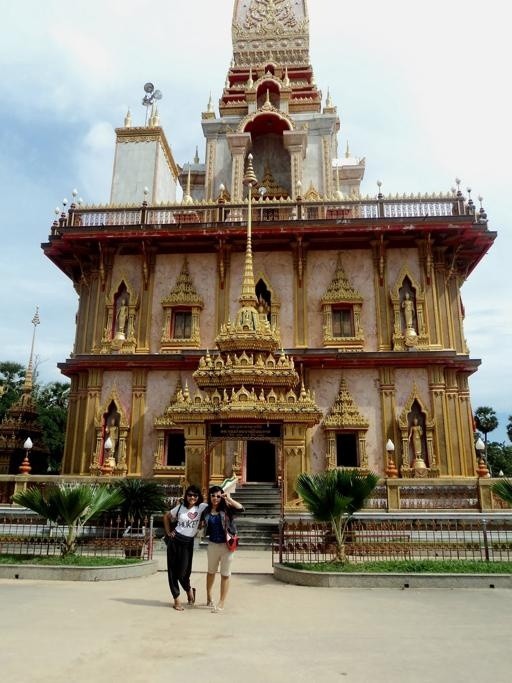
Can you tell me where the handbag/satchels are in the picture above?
[226,533,238,551]
[163,520,178,545]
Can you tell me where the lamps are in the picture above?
[377,178,383,199]
[100,437,113,475]
[218,183,225,204]
[451,176,487,220]
[296,179,302,200]
[19,436,32,474]
[385,438,399,479]
[53,189,83,226]
[475,437,490,478]
[142,186,149,207]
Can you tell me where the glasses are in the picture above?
[210,493,221,498]
[187,493,198,498]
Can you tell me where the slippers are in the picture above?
[211,606,224,613]
[174,605,183,610]
[188,588,196,607]
[207,602,214,607]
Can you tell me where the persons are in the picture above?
[163,485,233,610]
[409,418,424,459]
[200,486,245,608]
[402,293,415,329]
[105,415,118,457]
[116,299,128,332]
